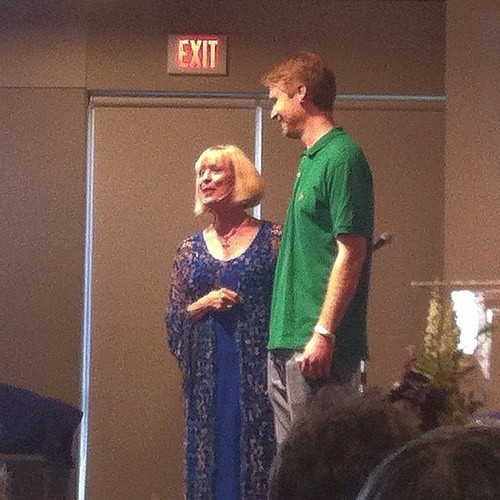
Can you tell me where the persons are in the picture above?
[260,52,374,451]
[165,144,283,500]
[270,385,500,500]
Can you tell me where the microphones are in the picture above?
[217,186,235,203]
[371,233,392,254]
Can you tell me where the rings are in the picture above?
[227,304,233,308]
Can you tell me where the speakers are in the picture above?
[0,382,84,455]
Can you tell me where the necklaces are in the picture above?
[212,214,253,249]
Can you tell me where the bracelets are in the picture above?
[314,325,337,341]
